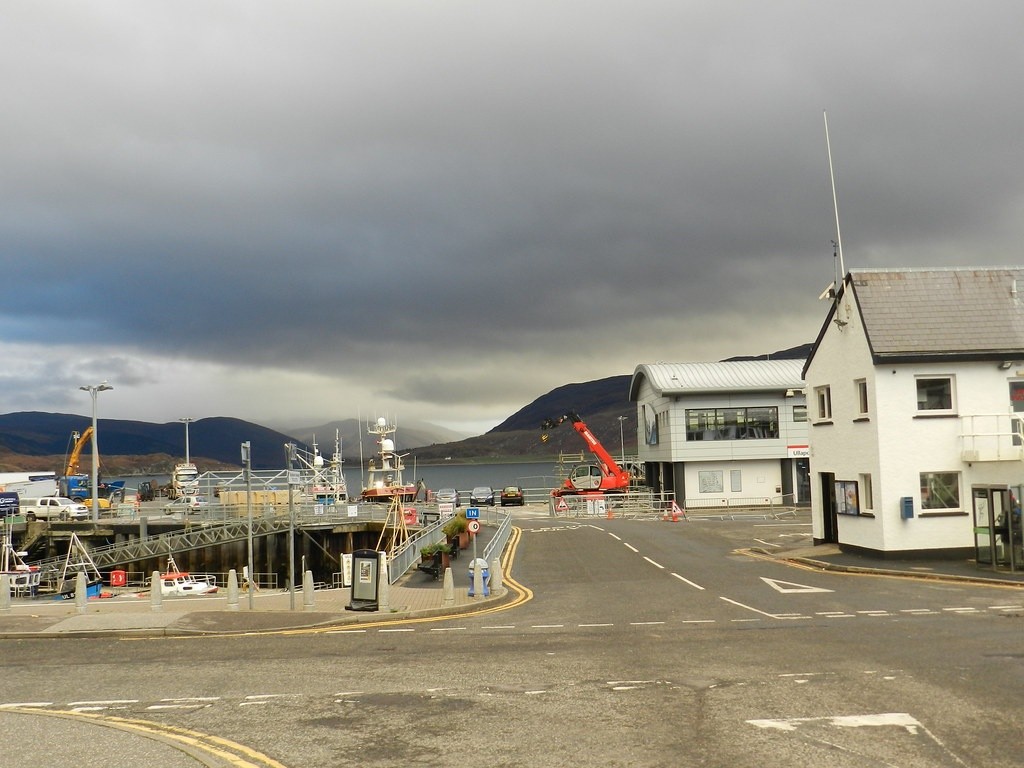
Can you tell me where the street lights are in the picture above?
[80,385,113,522]
[617,416,628,466]
[179,417,194,464]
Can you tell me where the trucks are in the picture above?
[0,475,90,503]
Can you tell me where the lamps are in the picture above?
[833,318,848,331]
[999,360,1013,368]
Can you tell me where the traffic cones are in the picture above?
[607,508,614,519]
[672,514,678,522]
[662,508,669,521]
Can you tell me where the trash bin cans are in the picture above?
[467,558,489,596]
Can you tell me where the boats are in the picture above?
[312,428,348,505]
[112,537,218,597]
[358,410,417,503]
[0,507,103,601]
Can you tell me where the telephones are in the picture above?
[997,511,1008,526]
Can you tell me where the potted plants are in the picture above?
[420,543,453,570]
[457,508,477,540]
[443,516,469,548]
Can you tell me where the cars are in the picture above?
[164,496,209,515]
[469,486,496,507]
[500,486,524,507]
[435,488,461,506]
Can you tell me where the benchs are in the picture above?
[418,559,438,580]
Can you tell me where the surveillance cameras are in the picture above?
[786,392,794,398]
[819,282,835,301]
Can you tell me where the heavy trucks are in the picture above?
[168,463,200,500]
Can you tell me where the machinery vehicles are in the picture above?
[66,428,100,476]
[541,412,630,509]
[137,482,155,502]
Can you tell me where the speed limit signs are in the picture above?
[468,520,480,533]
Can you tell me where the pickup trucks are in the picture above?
[19,497,89,522]
